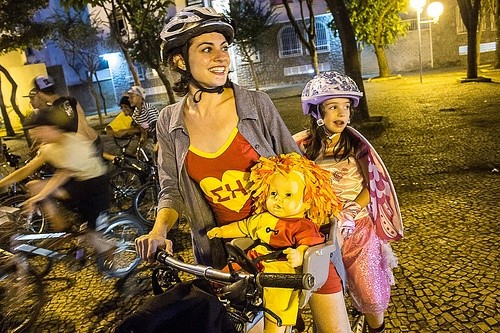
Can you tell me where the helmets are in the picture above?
[301,71,363,116]
[160,5,234,67]
[127,86,146,99]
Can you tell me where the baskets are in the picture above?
[108,311,244,333]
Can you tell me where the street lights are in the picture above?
[409,0,444,83]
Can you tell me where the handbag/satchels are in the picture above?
[115,266,239,333]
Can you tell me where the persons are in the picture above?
[0,76,163,267]
[134,3,353,333]
[207,151,345,333]
[289,71,404,333]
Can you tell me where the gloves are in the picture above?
[338,199,361,238]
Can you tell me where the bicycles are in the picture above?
[133,137,161,226]
[0,143,72,233]
[106,130,146,217]
[0,200,145,333]
[111,238,369,333]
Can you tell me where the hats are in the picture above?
[23,88,39,97]
[33,75,55,91]
